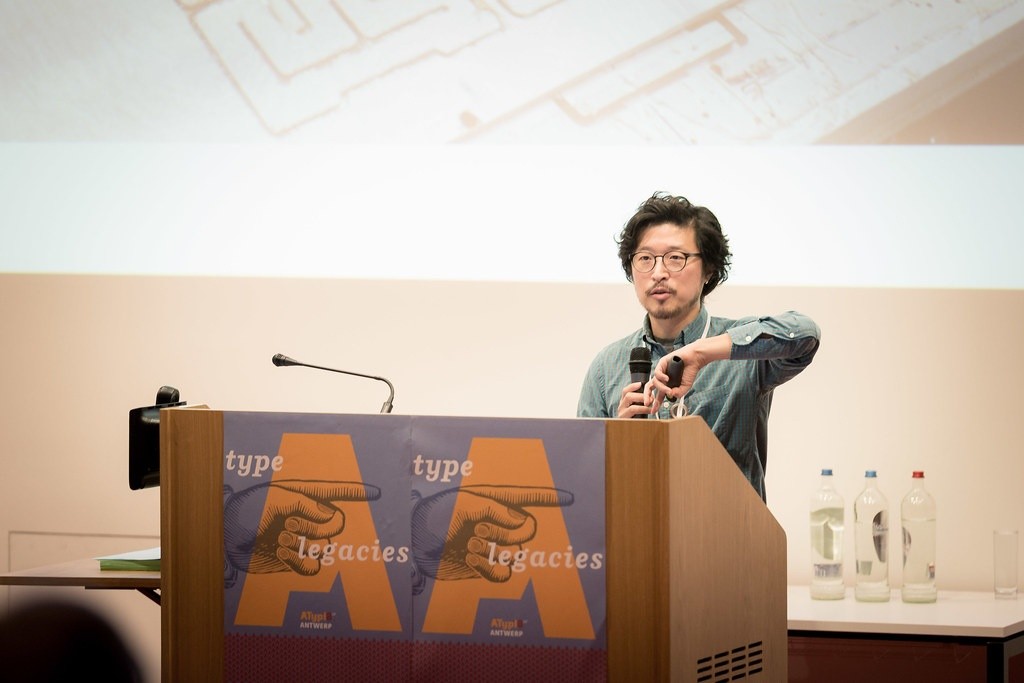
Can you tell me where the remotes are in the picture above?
[666,356,684,403]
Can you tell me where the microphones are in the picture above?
[629,347,653,420]
[272,353,394,414]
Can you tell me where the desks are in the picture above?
[0,560,1024,683]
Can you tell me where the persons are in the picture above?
[577,189,822,505]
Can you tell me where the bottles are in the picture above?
[899,472,939,603]
[854,470,891,602]
[809,468,846,600]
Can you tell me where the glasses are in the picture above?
[629,250,701,273]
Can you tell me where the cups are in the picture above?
[994,529,1018,599]
